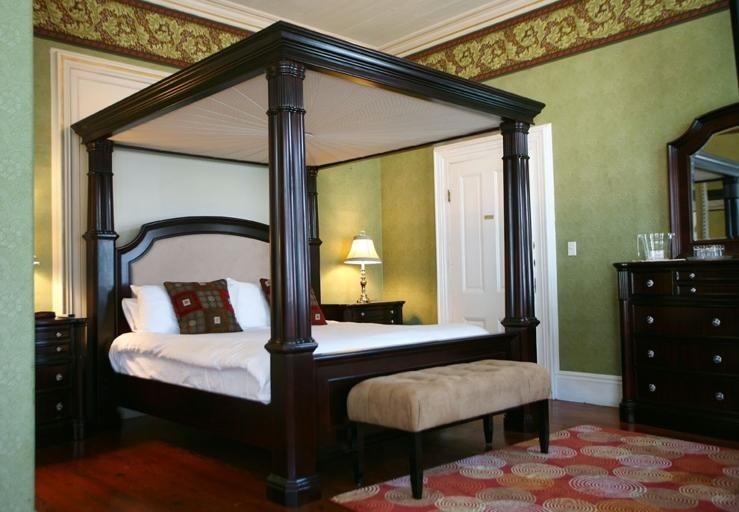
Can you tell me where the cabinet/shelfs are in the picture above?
[613,260,737,439]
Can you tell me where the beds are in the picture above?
[70,21,545,507]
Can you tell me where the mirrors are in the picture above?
[666,102,738,259]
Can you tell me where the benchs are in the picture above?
[346,358,553,500]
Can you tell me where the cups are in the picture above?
[693,245,725,257]
[632,231,679,263]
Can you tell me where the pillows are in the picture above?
[259,277,327,325]
[130,284,180,334]
[120,298,137,333]
[164,278,243,335]
[227,276,272,330]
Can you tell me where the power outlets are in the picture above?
[567,241,576,256]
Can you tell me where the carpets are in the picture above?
[330,425,734,511]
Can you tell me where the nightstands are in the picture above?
[322,301,407,326]
[35,317,93,465]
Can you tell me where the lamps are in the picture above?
[344,231,381,303]
